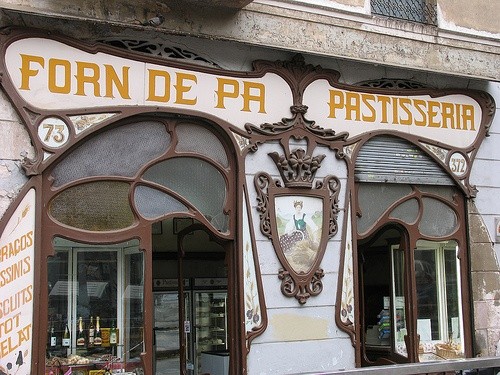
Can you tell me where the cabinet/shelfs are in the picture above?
[196,296,227,352]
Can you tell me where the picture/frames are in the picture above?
[152,221,163,235]
[173,218,194,234]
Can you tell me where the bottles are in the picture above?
[94,316,102,347]
[62,323,70,348]
[47,327,56,351]
[110,319,117,355]
[88,316,94,348]
[76,316,85,349]
